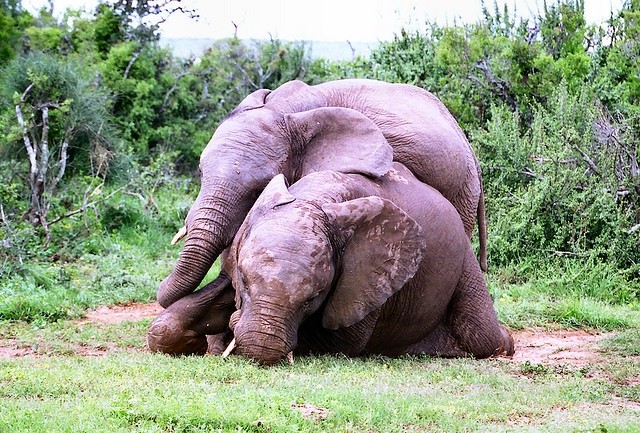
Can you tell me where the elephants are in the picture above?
[147,161,516,370]
[156,76,488,309]
